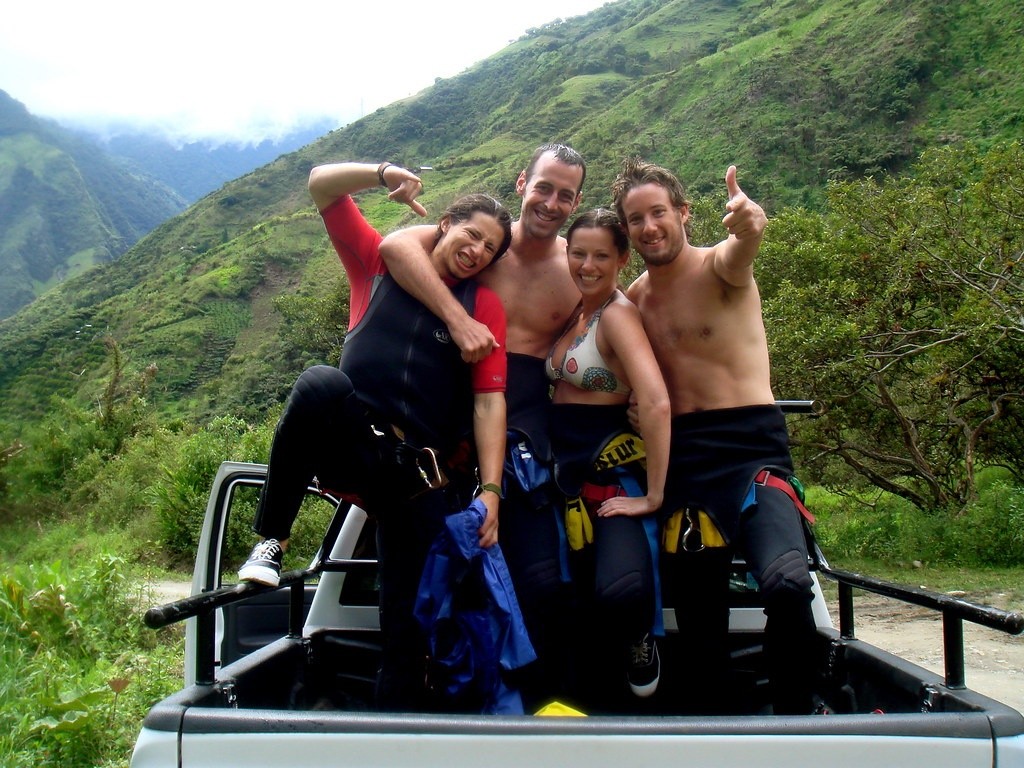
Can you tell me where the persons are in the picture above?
[240,160,506,716]
[379,143,820,715]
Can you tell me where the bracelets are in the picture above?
[481,483,503,500]
[379,162,392,186]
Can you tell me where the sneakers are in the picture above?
[626,632,661,696]
[238,539,284,586]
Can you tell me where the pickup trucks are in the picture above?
[128,460,1024,767]
